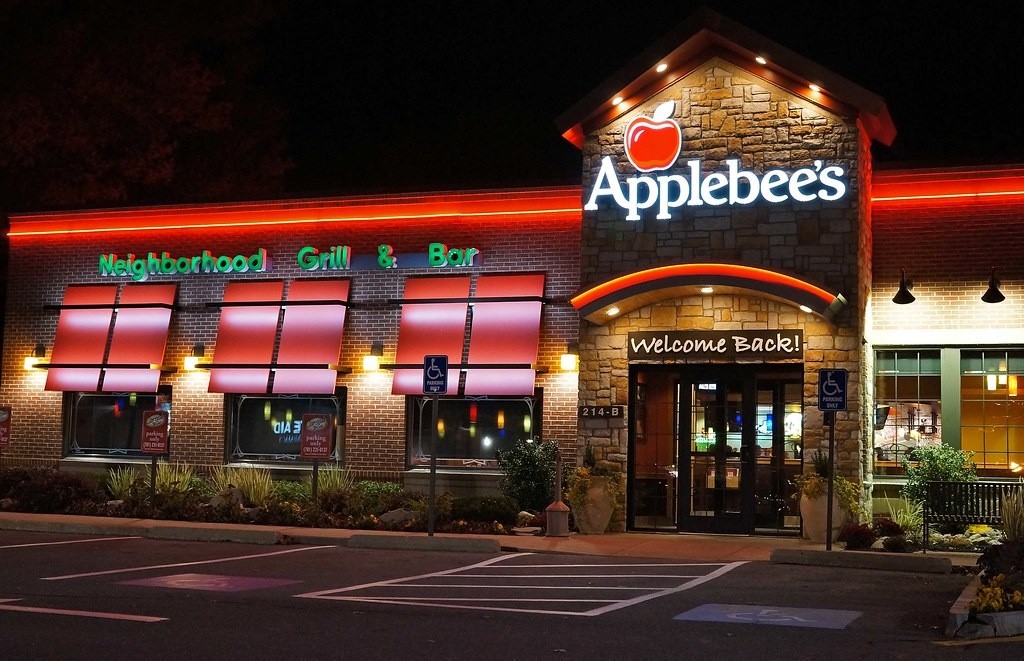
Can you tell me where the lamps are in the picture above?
[981,266,1005,303]
[363,344,384,371]
[892,268,916,305]
[560,343,579,371]
[24,347,46,370]
[184,345,204,370]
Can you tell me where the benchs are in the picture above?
[922,480,1024,554]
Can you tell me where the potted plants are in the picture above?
[564,440,623,535]
[792,448,873,544]
